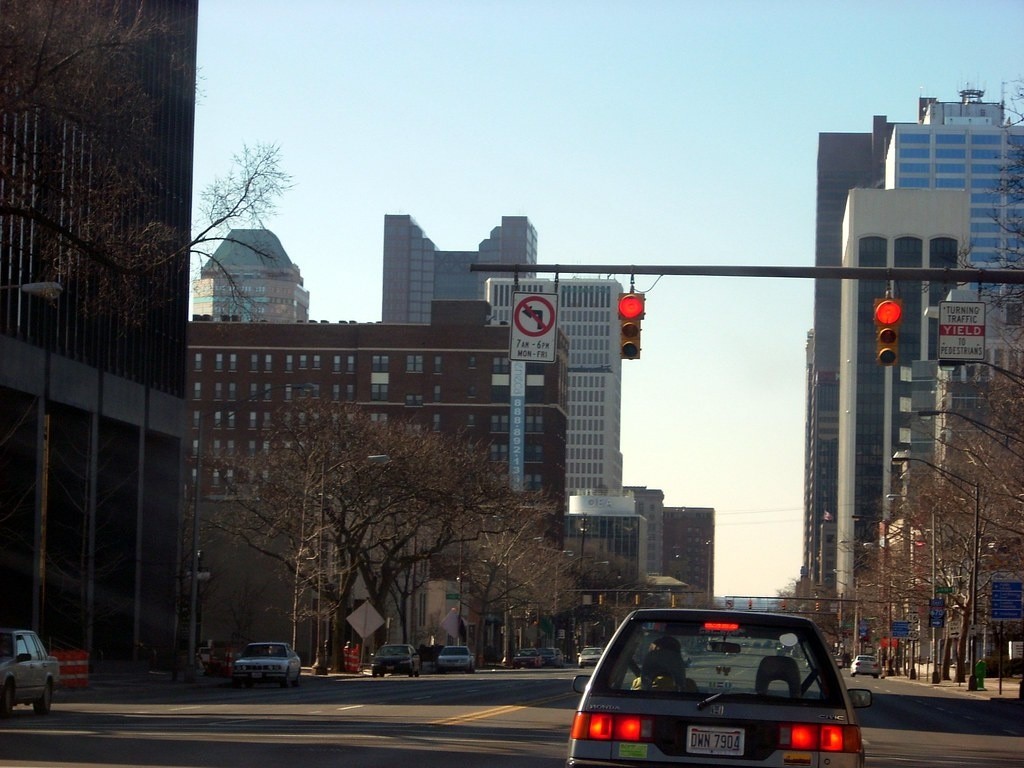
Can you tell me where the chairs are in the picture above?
[640,650,687,693]
[755,656,802,698]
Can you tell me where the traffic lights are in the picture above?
[749,603,753,611]
[875,298,905,367]
[782,603,786,611]
[616,292,646,360]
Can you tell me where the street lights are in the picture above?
[307,454,389,674]
[833,569,859,661]
[187,382,315,685]
[456,515,498,644]
[887,493,941,684]
[863,543,895,677]
[891,450,983,693]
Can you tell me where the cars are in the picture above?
[565,608,873,768]
[371,644,421,677]
[513,648,542,668]
[438,646,477,675]
[577,648,604,669]
[850,655,880,678]
[0,630,60,718]
[232,643,302,689]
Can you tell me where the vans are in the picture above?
[197,647,211,666]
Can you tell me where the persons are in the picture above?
[842,653,850,667]
[881,652,887,678]
[777,645,781,655]
[632,637,699,693]
[804,657,808,667]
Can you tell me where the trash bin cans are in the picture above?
[975,659,987,691]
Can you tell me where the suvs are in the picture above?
[540,648,566,668]
